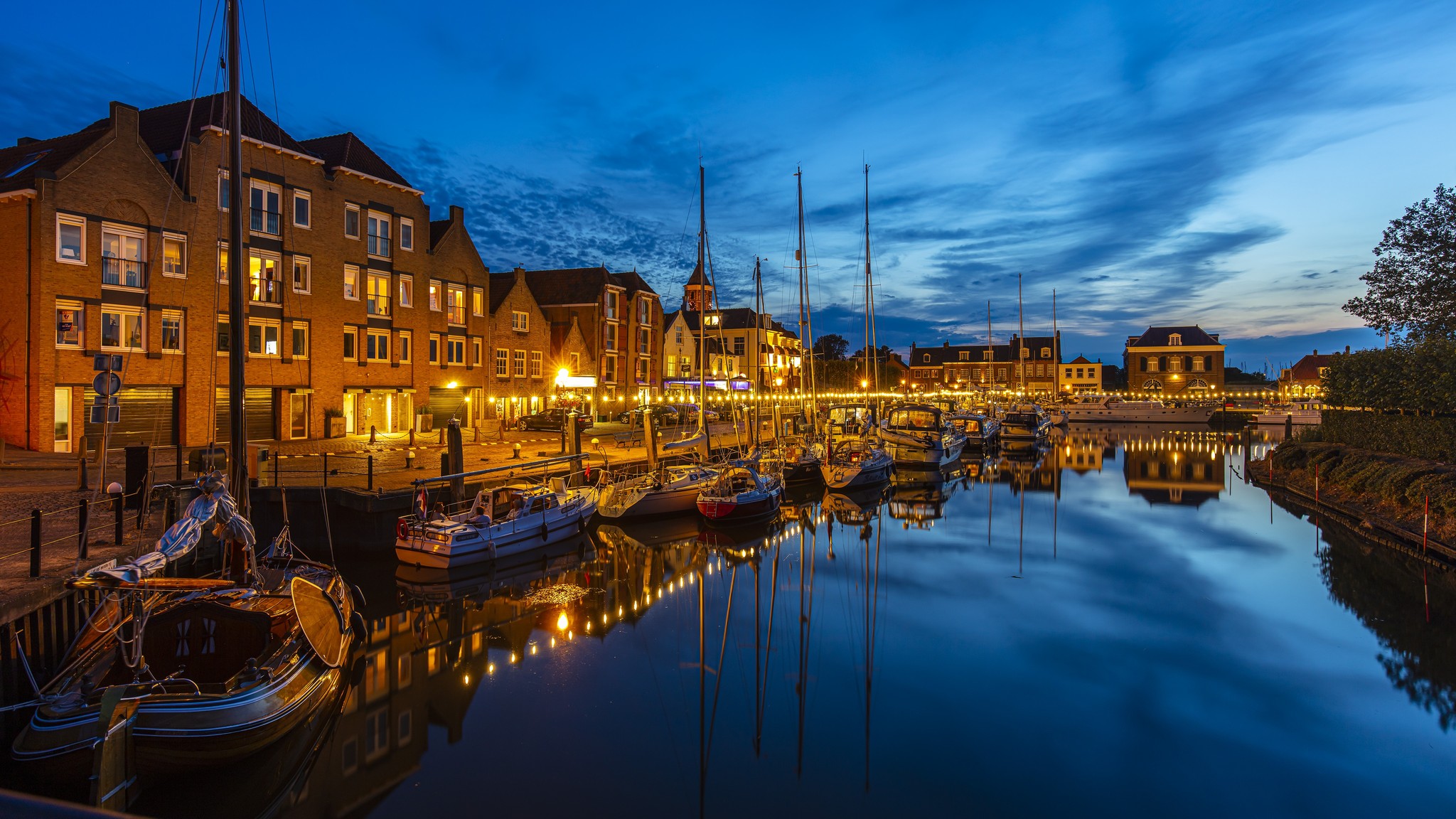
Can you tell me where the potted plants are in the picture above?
[414,403,434,433]
[320,405,348,438]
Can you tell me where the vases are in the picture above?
[1226,407,1234,409]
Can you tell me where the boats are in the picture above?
[1039,397,1222,423]
[889,466,1056,530]
[1247,398,1329,425]
[1266,404,1292,410]
[394,534,608,658]
[820,397,1056,467]
[696,466,785,528]
[396,452,602,568]
[695,516,787,553]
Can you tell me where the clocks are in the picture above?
[708,302,712,310]
[689,301,696,310]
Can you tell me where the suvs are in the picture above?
[658,403,702,425]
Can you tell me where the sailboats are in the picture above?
[2,1,374,795]
[601,146,785,518]
[1048,287,1069,430]
[620,496,885,819]
[747,154,899,493]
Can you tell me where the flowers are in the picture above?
[1225,404,1234,407]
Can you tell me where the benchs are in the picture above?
[613,431,644,448]
[1246,404,1260,409]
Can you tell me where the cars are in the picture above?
[617,404,679,426]
[698,405,720,423]
[515,407,593,433]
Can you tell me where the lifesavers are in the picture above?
[397,588,408,609]
[397,519,409,539]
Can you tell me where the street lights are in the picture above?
[1066,385,1070,393]
[955,385,958,393]
[902,380,905,392]
[912,384,916,395]
[1211,385,1216,404]
